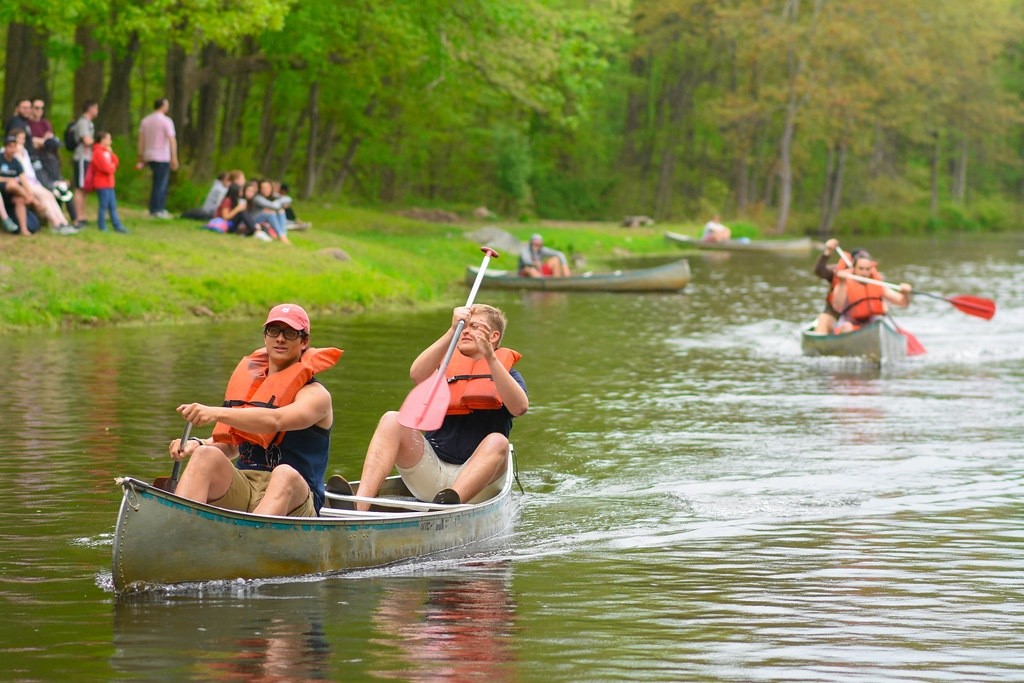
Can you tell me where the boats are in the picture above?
[468,259,691,293]
[801,318,908,368]
[112,443,514,592]
[665,231,814,259]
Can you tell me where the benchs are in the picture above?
[318,490,478,518]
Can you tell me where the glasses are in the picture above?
[266,325,301,340]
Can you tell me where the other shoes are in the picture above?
[254,230,273,242]
[3,217,18,233]
[325,474,357,511]
[50,223,79,235]
[145,209,175,221]
[427,488,460,512]
[294,222,312,232]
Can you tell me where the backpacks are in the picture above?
[83,161,93,192]
[64,122,82,153]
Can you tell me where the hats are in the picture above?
[534,239,542,245]
[4,135,17,147]
[54,182,73,202]
[263,303,310,335]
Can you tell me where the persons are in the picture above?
[136,97,179,218]
[201,170,313,243]
[168,304,333,516]
[0,98,126,237]
[325,303,528,512]
[812,238,912,334]
[518,233,571,277]
[703,213,730,242]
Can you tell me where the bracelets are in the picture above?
[137,154,143,162]
[189,436,204,445]
[15,177,20,182]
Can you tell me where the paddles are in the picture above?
[840,272,997,320]
[397,246,499,432]
[153,406,193,494]
[836,246,927,356]
